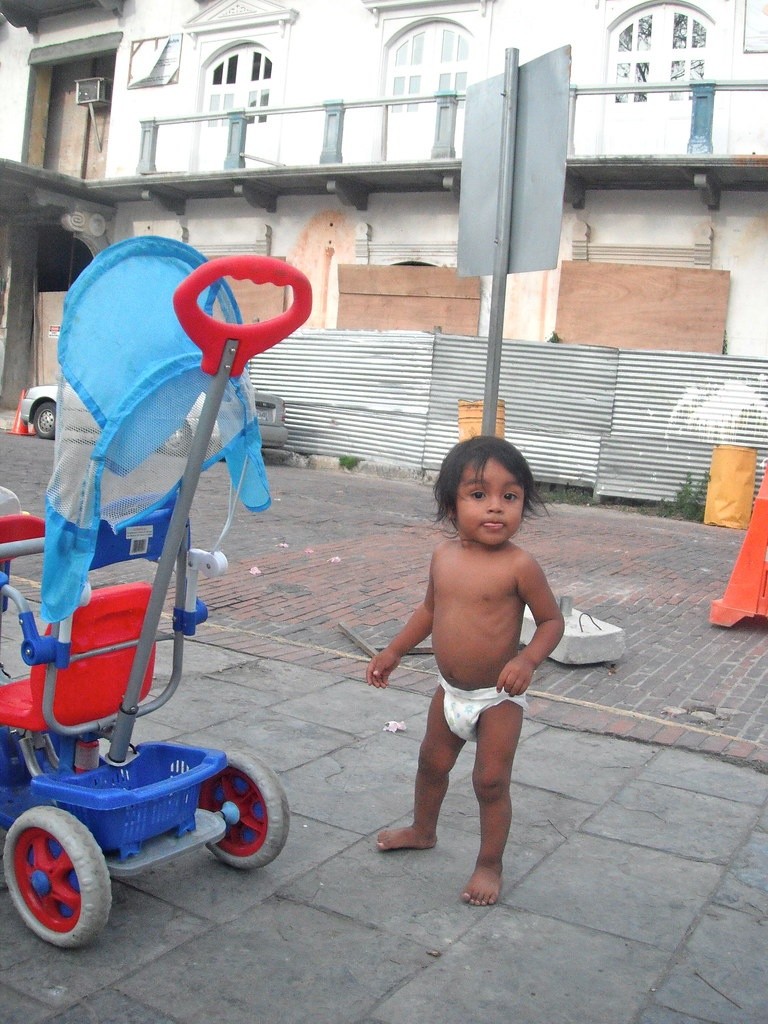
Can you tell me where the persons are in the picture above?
[364,434,566,906]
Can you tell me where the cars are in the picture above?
[20,379,289,456]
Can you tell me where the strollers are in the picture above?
[1,231,312,953]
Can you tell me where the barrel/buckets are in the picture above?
[457,400,505,442]
[704,445,758,530]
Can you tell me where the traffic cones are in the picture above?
[7,388,36,436]
[708,451,768,628]
